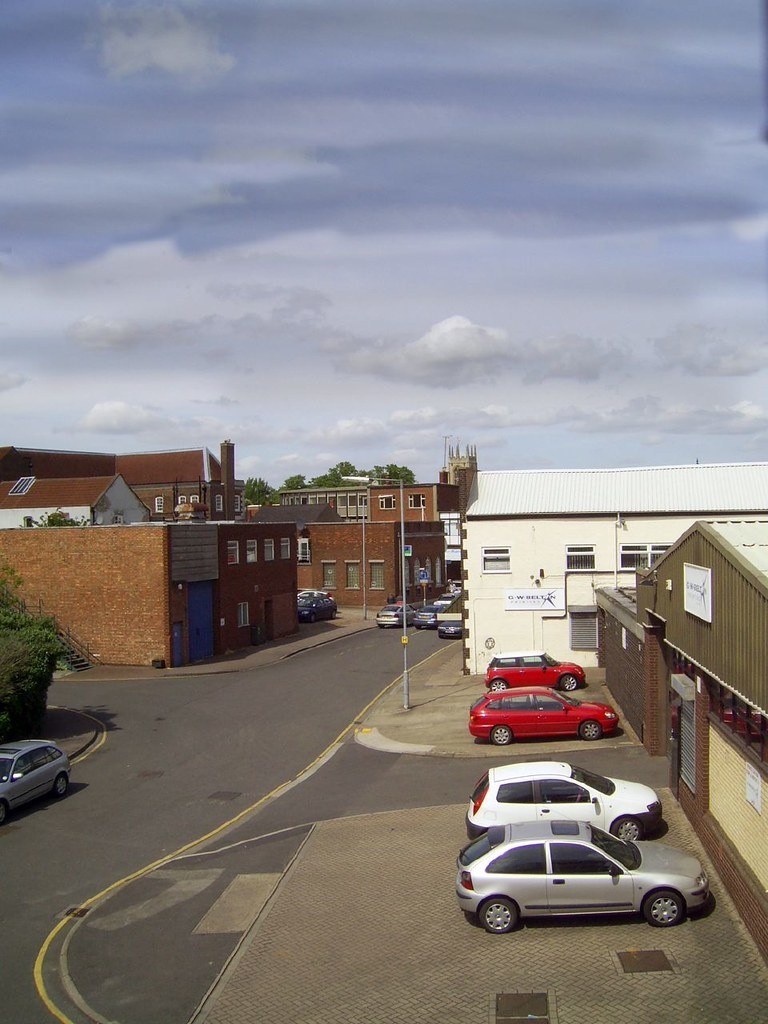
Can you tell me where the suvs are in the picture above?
[465,761,663,842]
[0,739,72,827]
[454,821,710,935]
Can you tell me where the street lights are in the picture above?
[342,476,411,710]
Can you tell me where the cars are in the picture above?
[484,651,587,692]
[438,621,464,639]
[296,591,338,623]
[433,579,463,607]
[377,603,419,629]
[469,685,620,747]
[412,605,444,630]
